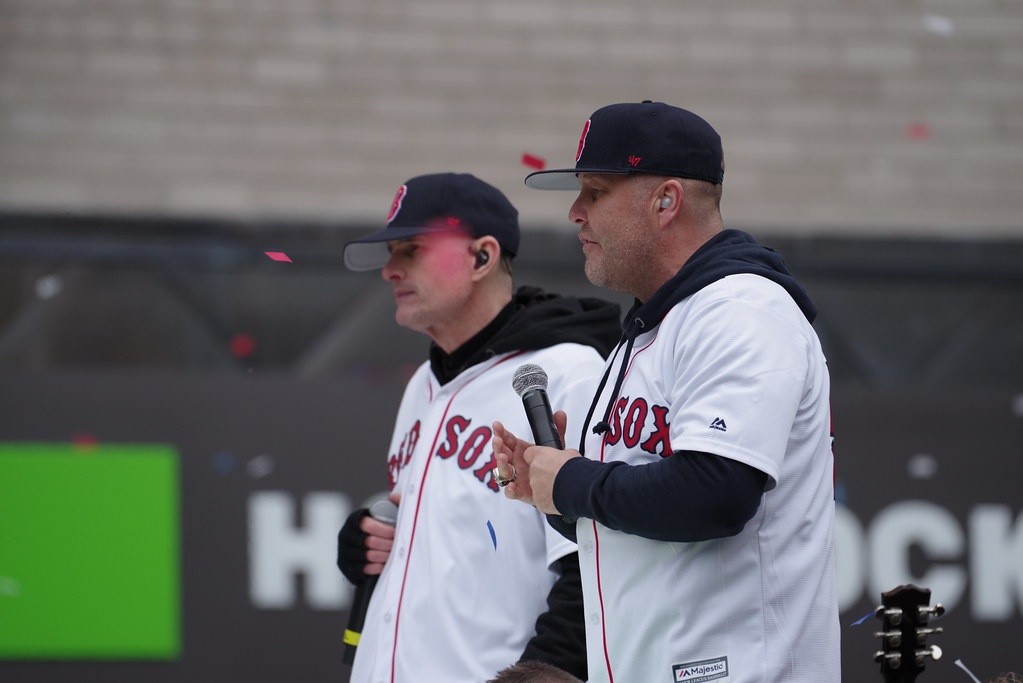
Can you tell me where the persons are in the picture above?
[336,171,623,683]
[491,100,843,683]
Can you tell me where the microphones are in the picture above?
[342,501,401,664]
[512,364,579,523]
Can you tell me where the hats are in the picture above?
[524,100,725,190]
[341,173,520,271]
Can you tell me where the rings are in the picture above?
[493,461,518,487]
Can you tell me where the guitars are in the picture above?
[872,583,945,683]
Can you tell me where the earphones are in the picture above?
[661,195,673,209]
[477,251,489,265]
